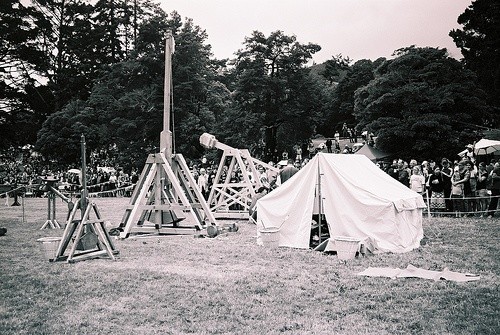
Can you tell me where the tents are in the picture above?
[256,152,427,257]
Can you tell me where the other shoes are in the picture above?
[422,209,494,219]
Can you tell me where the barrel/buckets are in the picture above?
[259,226,280,251]
[333,236,361,262]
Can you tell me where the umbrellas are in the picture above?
[457,137,500,164]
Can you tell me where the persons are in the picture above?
[0,144,154,198]
[293,122,390,171]
[484,119,490,132]
[462,143,477,165]
[374,156,500,219]
[490,119,496,132]
[165,153,299,211]
